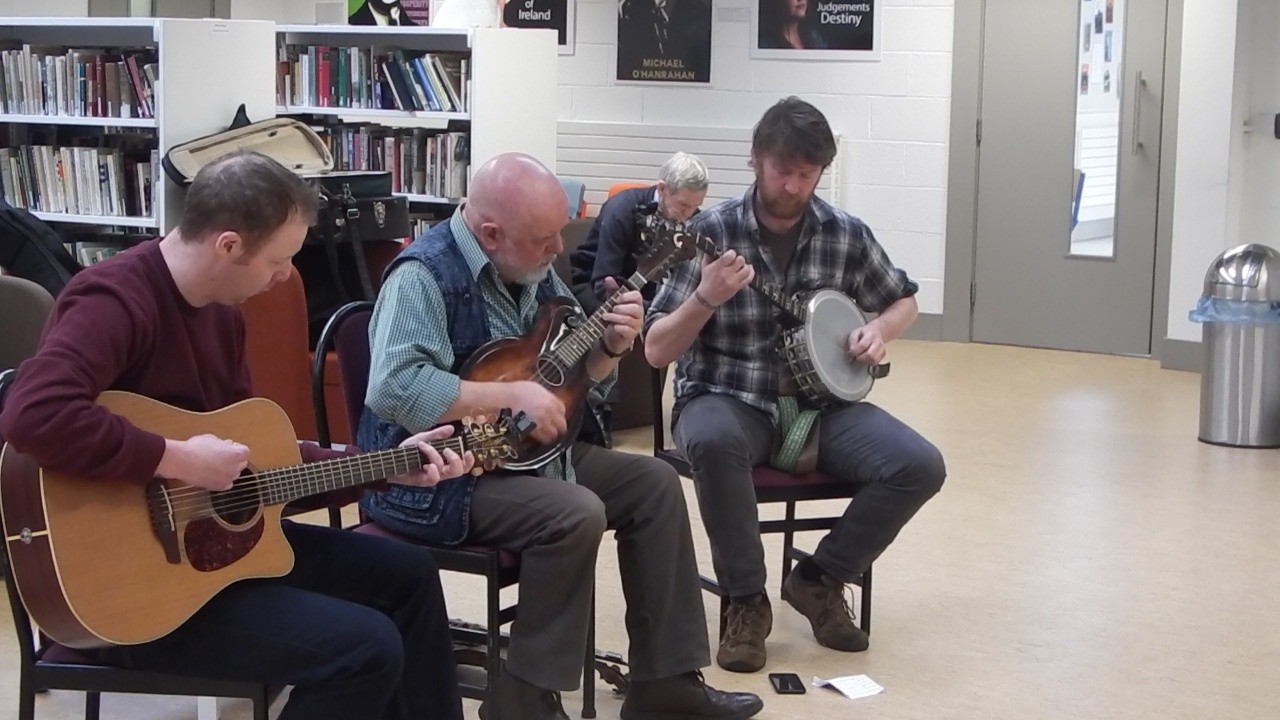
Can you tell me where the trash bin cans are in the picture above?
[1197,243,1280,448]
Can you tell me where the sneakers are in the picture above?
[780,557,869,652]
[717,587,773,673]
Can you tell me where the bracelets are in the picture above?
[694,289,719,311]
[600,335,634,358]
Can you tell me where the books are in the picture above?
[0,41,161,266]
[277,33,471,245]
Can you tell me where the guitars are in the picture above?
[636,200,877,410]
[0,390,520,651]
[459,226,698,472]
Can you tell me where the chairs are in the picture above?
[0,183,871,720]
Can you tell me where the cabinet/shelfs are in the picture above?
[271,25,558,237]
[0,16,276,266]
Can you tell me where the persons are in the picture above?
[568,150,711,451]
[641,93,947,674]
[357,153,764,720]
[0,152,464,720]
[758,0,827,49]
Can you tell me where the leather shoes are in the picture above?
[620,667,764,719]
[477,662,569,720]
